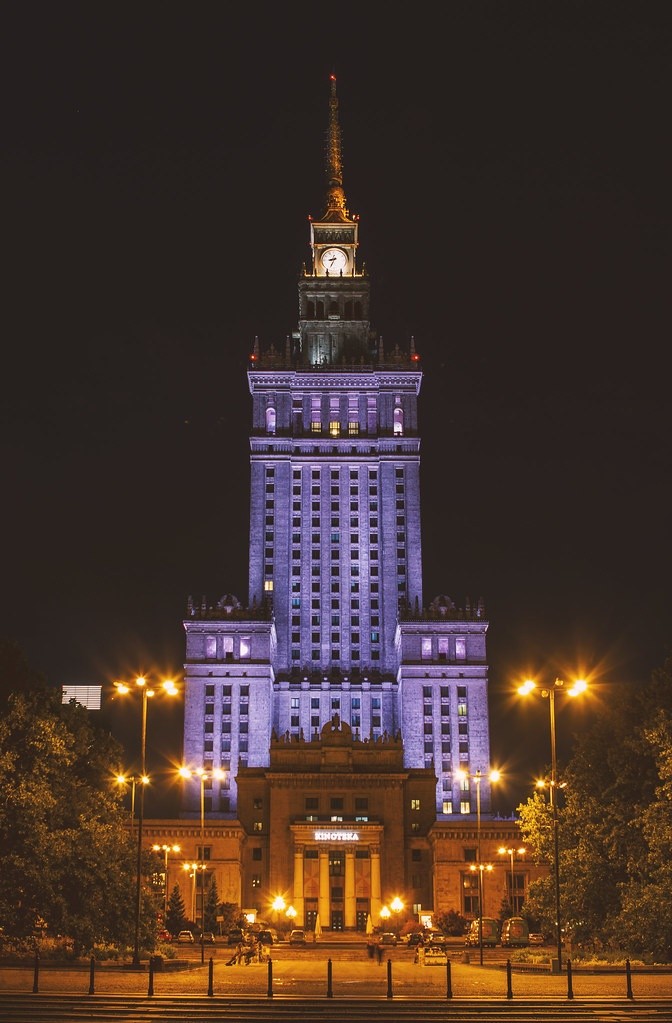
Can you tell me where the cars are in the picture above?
[528,934,543,946]
[379,933,397,946]
[408,934,424,946]
[199,932,215,944]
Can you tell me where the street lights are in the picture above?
[497,848,525,917]
[536,779,567,807]
[517,677,590,970]
[469,865,493,916]
[111,674,179,965]
[184,863,206,922]
[153,843,179,922]
[119,775,149,831]
[454,768,500,965]
[179,767,223,933]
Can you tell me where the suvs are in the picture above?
[157,930,172,943]
[178,931,194,944]
[290,930,306,945]
[258,930,273,945]
[429,933,446,947]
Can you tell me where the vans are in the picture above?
[501,917,529,948]
[228,928,247,944]
[466,917,499,947]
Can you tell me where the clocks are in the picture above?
[321,249,347,271]
